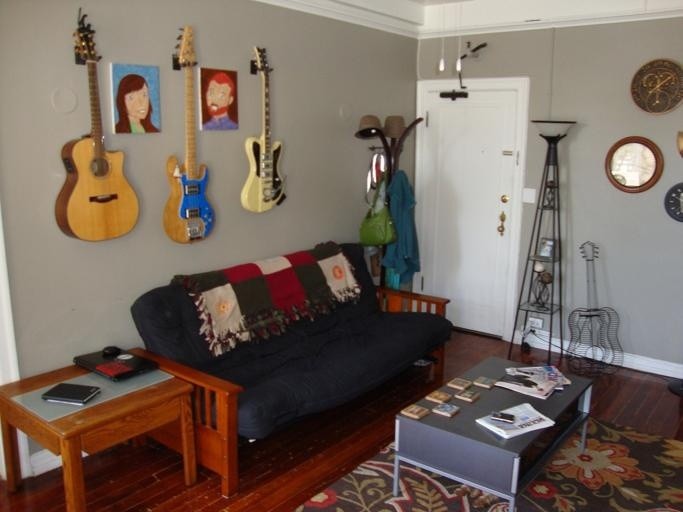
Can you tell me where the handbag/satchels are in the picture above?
[360,206,396,245]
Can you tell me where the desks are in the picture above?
[0,365,197,511]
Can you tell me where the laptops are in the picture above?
[73,349,159,382]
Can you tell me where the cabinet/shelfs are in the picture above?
[507,134,568,366]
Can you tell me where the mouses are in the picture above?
[103,346,120,356]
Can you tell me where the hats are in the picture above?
[382,116,410,139]
[355,115,382,139]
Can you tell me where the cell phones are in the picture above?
[490,410,515,424]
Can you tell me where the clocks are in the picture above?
[630,58,683,114]
[664,182,683,222]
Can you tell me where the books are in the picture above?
[494,365,572,400]
[41,383,101,404]
[474,402,557,440]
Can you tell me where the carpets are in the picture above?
[294,415,683,511]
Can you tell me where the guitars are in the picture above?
[240,45,287,213]
[567,241,624,377]
[54,6,139,241]
[163,25,213,243]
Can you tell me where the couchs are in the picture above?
[129,241,450,499]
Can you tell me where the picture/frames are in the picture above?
[605,136,664,193]
